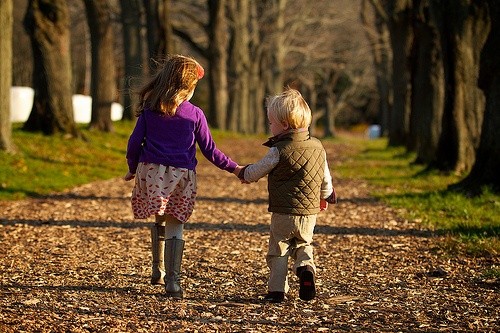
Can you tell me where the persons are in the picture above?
[123,55,246,299]
[237,88,337,304]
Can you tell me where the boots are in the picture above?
[150,226,165,285]
[163,235,185,297]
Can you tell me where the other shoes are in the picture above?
[264,292,284,303]
[299,265,316,301]
